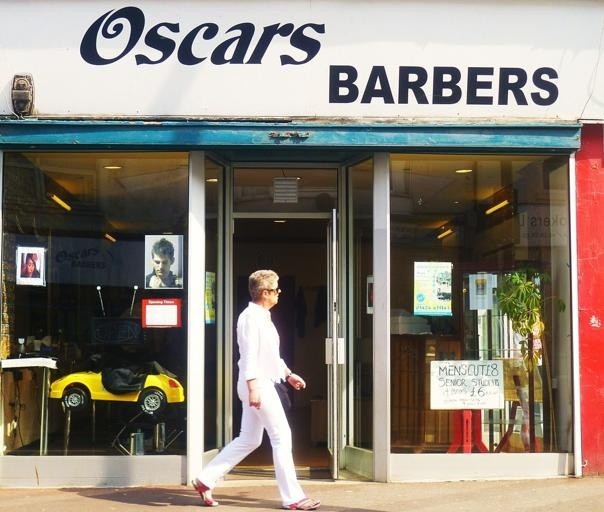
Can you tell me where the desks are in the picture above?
[0,358,58,455]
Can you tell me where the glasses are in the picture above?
[264,288,280,295]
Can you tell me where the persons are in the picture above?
[20,253,39,278]
[145,238,181,288]
[190,267,322,510]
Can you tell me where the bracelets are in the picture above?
[284,373,292,381]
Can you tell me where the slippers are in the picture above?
[192,477,219,507]
[282,497,321,510]
